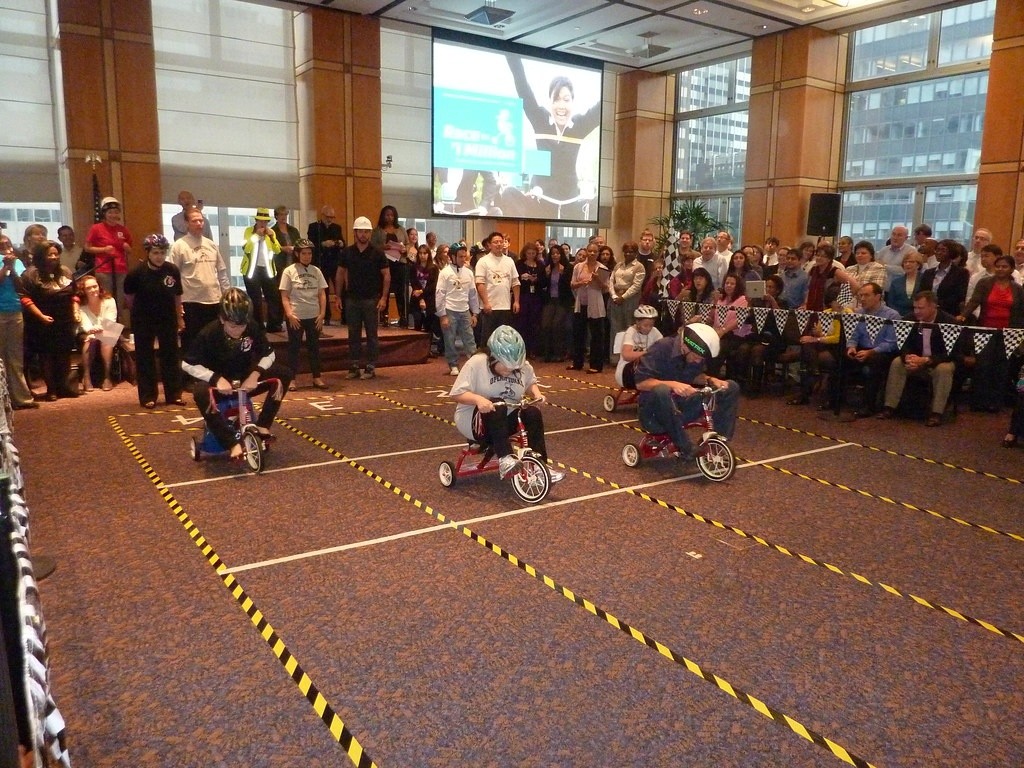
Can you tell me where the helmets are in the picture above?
[100,196,122,212]
[294,239,315,251]
[448,243,468,254]
[143,233,170,250]
[470,245,487,254]
[221,287,252,324]
[487,324,526,369]
[635,305,658,318]
[684,323,720,357]
[354,217,373,229]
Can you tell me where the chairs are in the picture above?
[776,360,965,422]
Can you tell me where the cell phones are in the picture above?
[197,200,203,208]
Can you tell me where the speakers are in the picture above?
[807,192,842,237]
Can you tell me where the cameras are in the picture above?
[387,155,392,159]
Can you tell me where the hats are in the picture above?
[255,207,271,221]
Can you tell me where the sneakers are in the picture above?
[549,469,566,483]
[499,454,523,482]
[450,366,459,376]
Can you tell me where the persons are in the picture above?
[182,288,293,461]
[434,54,600,223]
[634,323,739,471]
[370,206,410,328]
[786,223,1024,446]
[448,325,567,487]
[645,231,815,397]
[335,217,391,380]
[241,207,281,333]
[307,206,347,325]
[170,208,232,393]
[270,205,301,327]
[406,228,573,376]
[84,199,134,321]
[1,224,118,405]
[614,305,664,388]
[565,228,657,374]
[125,236,188,407]
[172,191,213,242]
[280,239,330,391]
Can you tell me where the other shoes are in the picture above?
[734,374,944,428]
[289,381,297,391]
[231,450,243,466]
[526,347,609,374]
[681,445,705,460]
[362,367,376,379]
[145,400,189,408]
[346,370,360,379]
[313,382,328,389]
[15,378,112,411]
[260,431,277,445]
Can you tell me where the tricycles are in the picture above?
[603,385,640,413]
[190,377,284,474]
[621,385,736,482]
[438,396,552,503]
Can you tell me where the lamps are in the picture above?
[463,0,514,26]
[626,30,671,60]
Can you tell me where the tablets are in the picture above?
[745,281,766,298]
